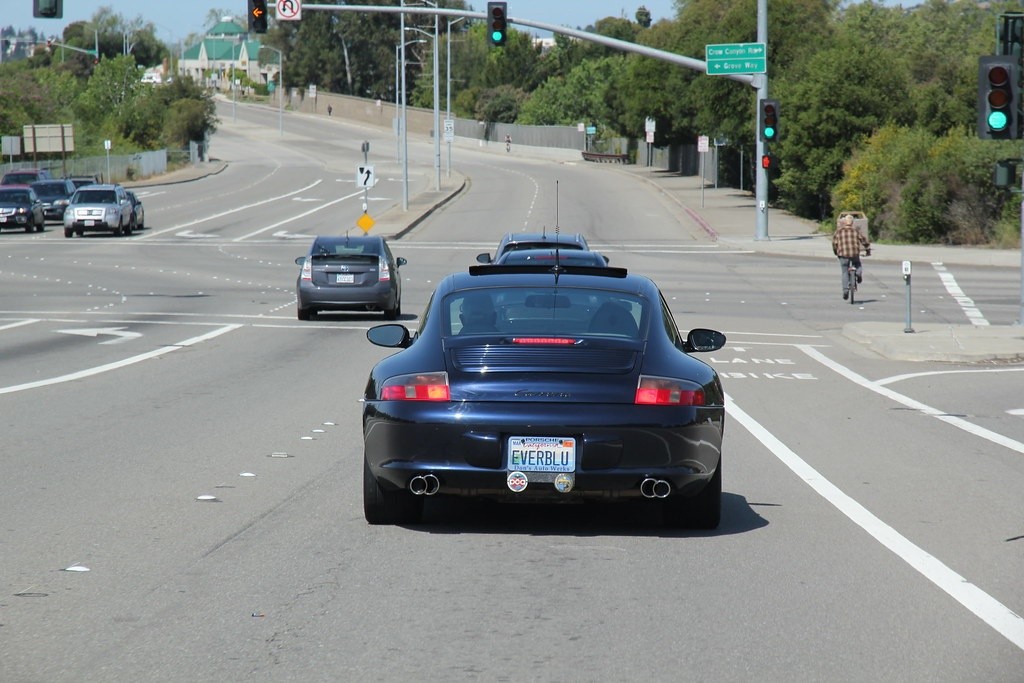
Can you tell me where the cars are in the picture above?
[294,235,408,321]
[459,248,632,331]
[0,187,45,233]
[475,232,610,264]
[0,170,105,220]
[63,184,144,238]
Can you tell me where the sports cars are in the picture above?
[360,262,727,529]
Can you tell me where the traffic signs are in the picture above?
[705,43,766,76]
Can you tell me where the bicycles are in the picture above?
[844,253,871,304]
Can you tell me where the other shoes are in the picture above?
[856,273,862,284]
[843,291,849,300]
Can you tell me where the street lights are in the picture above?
[259,45,283,141]
[395,39,427,164]
[404,27,436,153]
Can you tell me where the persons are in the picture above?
[832,214,872,300]
[458,293,500,334]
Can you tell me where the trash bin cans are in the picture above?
[837,211,870,252]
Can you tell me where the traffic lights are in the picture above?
[93,58,99,65]
[248,0,268,34]
[976,53,1019,140]
[47,41,50,47]
[762,155,777,169]
[487,2,508,45]
[759,98,779,143]
